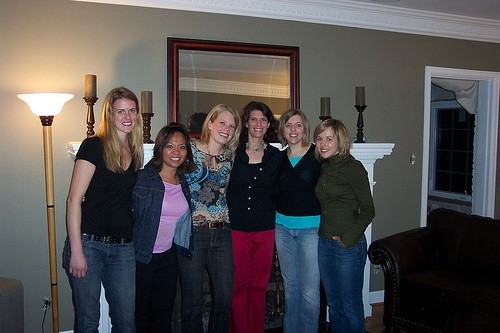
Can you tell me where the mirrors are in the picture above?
[167,36,300,141]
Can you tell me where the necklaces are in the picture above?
[206,141,224,171]
[246,142,266,153]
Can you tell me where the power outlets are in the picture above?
[41,296,53,312]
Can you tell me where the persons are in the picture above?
[62,87,144,333]
[82,122,198,333]
[226,101,279,333]
[176,104,241,333]
[313,118,375,333]
[275,110,323,333]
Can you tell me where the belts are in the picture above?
[89,234,133,244]
[193,220,228,229]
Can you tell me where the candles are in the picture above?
[141,90,152,113]
[355,87,366,105]
[84,74,97,98]
[320,97,330,116]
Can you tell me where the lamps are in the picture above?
[17,93,75,333]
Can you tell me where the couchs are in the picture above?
[368,208,500,333]
